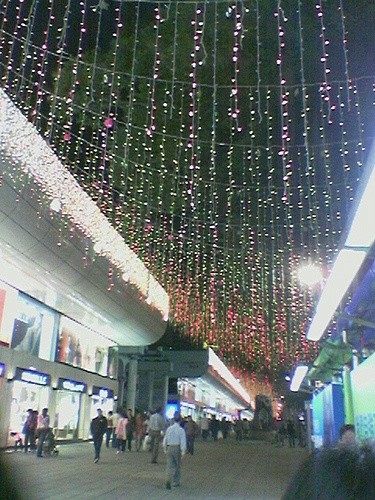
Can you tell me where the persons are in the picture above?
[90,408,374,500]
[11,408,50,457]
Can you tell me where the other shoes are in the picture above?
[165,483,171,489]
[172,484,180,487]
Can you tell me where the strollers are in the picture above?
[40,428,58,457]
